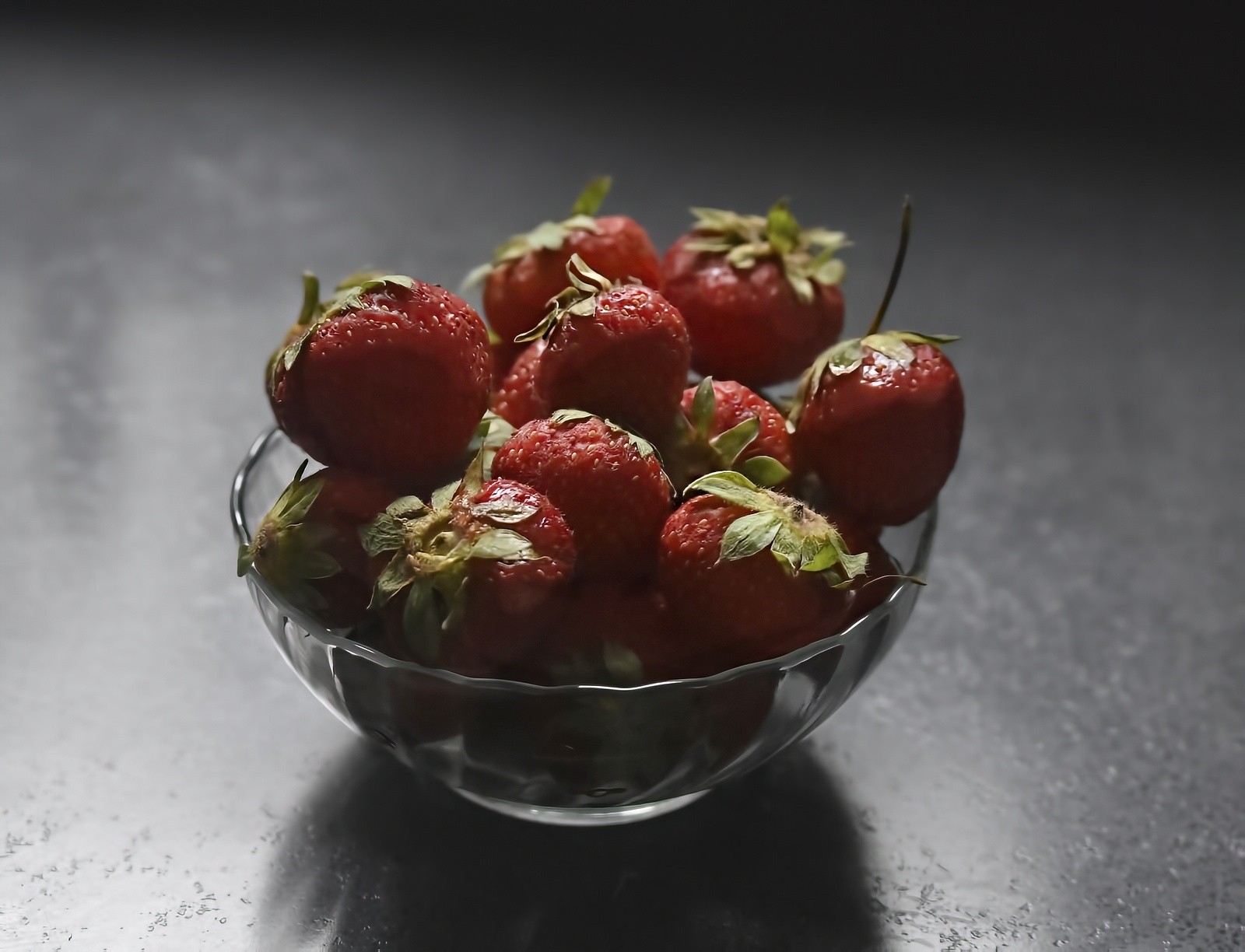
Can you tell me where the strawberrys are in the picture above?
[239,175,966,755]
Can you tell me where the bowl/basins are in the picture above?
[229,423,949,829]
